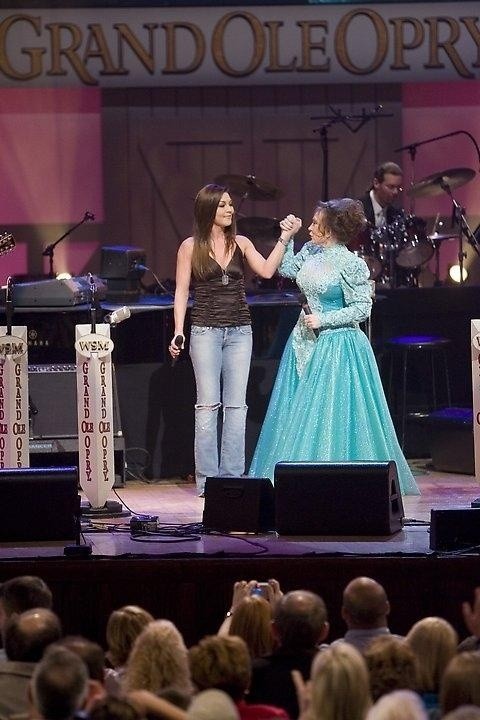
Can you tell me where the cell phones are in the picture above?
[251,582,269,596]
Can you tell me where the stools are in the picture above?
[381,332,456,457]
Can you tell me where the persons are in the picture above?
[218,576,428,718]
[103,605,289,718]
[278,237,288,246]
[277,193,376,464]
[353,161,405,254]
[167,180,303,498]
[405,588,479,718]
[0,576,106,719]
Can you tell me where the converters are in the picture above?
[130,515,159,532]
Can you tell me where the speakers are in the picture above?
[203,477,273,532]
[274,459,404,536]
[429,406,474,474]
[0,466,79,542]
[430,508,480,550]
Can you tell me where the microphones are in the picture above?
[297,293,319,338]
[171,335,183,367]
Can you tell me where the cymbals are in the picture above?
[234,216,281,238]
[214,173,283,202]
[429,231,456,241]
[408,167,477,198]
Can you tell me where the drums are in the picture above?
[396,238,435,267]
